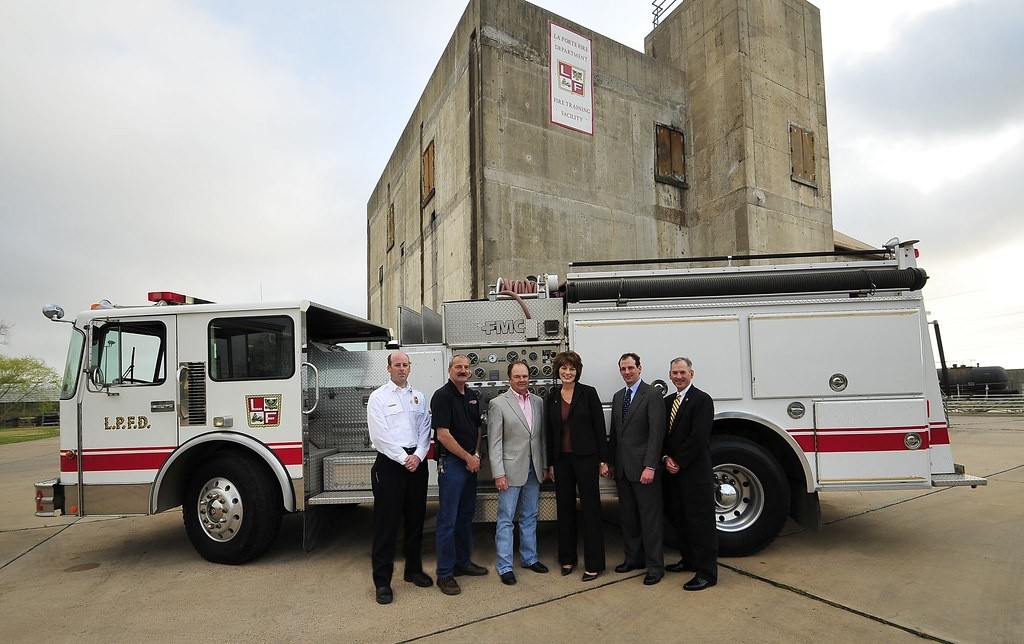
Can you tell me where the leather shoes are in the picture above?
[683,576,714,591]
[562,565,573,576]
[523,561,548,573]
[615,561,633,573]
[501,571,517,585]
[582,573,597,580]
[665,562,685,572]
[643,572,664,585]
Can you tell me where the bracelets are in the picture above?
[662,455,667,462]
[599,462,606,466]
[474,453,481,459]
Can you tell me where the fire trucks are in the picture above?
[33,236,987,557]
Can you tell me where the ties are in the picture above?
[622,389,633,427]
[669,394,681,432]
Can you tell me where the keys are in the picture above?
[436,463,445,473]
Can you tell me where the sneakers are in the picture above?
[404,572,433,587]
[454,562,488,576]
[376,585,393,604]
[436,576,461,595]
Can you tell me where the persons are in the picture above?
[367,352,434,605]
[543,350,610,582]
[430,354,489,596]
[488,361,548,585]
[610,353,667,586]
[664,357,718,590]
